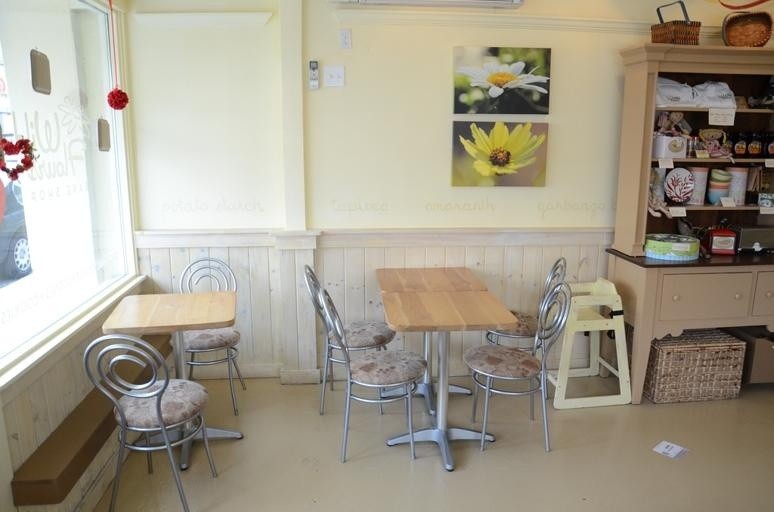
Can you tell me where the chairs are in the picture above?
[302,262,399,417]
[313,283,430,464]
[461,283,572,453]
[80,330,217,511]
[482,256,568,401]
[174,258,246,418]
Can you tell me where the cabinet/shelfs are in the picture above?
[608,44,774,256]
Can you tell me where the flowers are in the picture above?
[0,139,38,180]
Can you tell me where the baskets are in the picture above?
[651,0,701,46]
[723,12,773,47]
[628,326,746,403]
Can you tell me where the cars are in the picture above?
[0,110,32,280]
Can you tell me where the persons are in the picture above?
[656,110,736,166]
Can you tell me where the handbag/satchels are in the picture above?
[656,76,737,108]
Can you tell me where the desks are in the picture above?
[603,248,773,404]
[102,294,244,469]
[374,268,519,473]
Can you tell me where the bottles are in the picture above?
[685,134,700,158]
[733,130,774,158]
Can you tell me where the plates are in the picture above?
[663,168,695,203]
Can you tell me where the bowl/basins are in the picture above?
[709,168,732,205]
